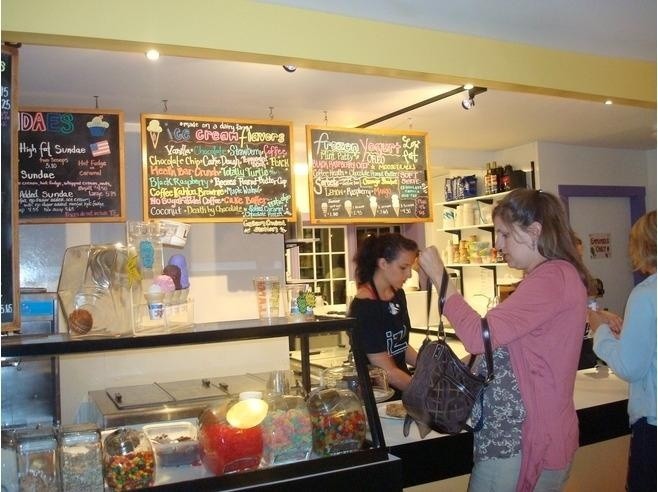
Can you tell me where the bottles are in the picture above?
[484,162,493,194]
[491,159,501,193]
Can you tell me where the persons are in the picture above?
[345,234,418,402]
[588,209,657,491]
[571,236,623,334]
[593,278,606,311]
[418,190,599,491]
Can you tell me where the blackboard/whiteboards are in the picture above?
[17,106,128,223]
[2,43,21,333]
[140,113,297,223]
[306,125,434,224]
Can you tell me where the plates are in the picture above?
[377,399,408,420]
[374,386,396,403]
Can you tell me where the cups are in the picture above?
[253,275,281,320]
[144,288,190,320]
[443,202,480,229]
[280,284,307,318]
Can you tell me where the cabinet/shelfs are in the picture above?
[436,160,535,338]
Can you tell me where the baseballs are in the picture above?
[68,309,93,336]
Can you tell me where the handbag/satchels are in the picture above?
[402,268,494,438]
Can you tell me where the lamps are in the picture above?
[462,87,486,110]
[284,65,296,73]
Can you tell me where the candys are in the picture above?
[2,433,61,489]
[262,409,313,463]
[103,449,156,489]
[199,421,263,473]
[311,411,367,456]
[58,440,105,491]
